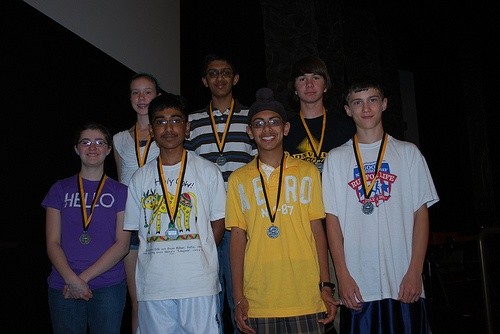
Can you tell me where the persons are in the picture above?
[281,56,355,334]
[40,122,132,334]
[114,74,163,334]
[322,79,439,334]
[184,57,258,334]
[123,94,229,334]
[227,99,337,334]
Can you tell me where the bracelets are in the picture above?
[233,295,245,309]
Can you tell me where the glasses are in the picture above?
[250,119,284,127]
[205,70,232,77]
[77,139,108,146]
[152,118,184,127]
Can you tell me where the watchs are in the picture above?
[319,281,336,296]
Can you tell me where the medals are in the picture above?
[166,227,178,240]
[317,164,324,173]
[268,226,280,238]
[80,232,91,245]
[216,157,225,164]
[362,203,375,215]
